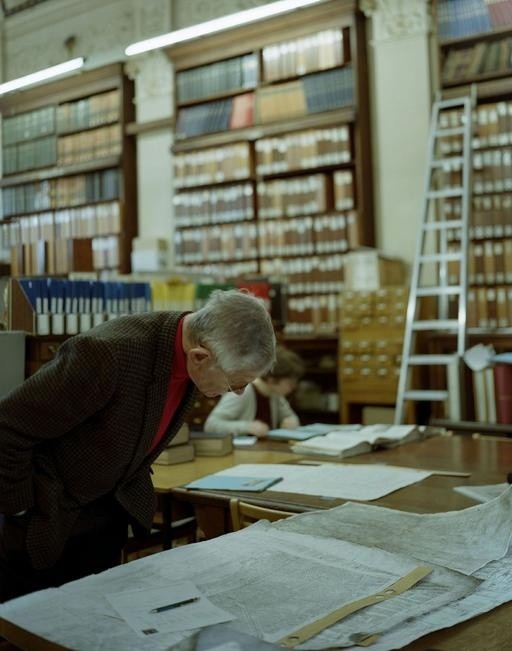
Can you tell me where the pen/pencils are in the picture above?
[150,597,201,614]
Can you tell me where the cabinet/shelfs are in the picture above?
[25,319,287,433]
[160,0,375,423]
[335,287,411,426]
[2,61,136,335]
[421,1,512,441]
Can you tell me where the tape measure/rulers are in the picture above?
[298,460,471,477]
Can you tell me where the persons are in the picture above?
[204,344,305,439]
[0,289,277,606]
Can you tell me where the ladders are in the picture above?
[394,96,470,425]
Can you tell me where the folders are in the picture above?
[19,279,152,314]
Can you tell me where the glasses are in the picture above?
[218,363,250,393]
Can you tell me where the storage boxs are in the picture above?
[342,249,408,291]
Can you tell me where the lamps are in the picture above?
[1,34,88,94]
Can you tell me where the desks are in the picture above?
[0,438,509,651]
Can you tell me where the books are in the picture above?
[172,26,359,335]
[439,38,512,327]
[265,422,419,458]
[459,344,512,428]
[1,89,120,276]
[16,276,270,336]
